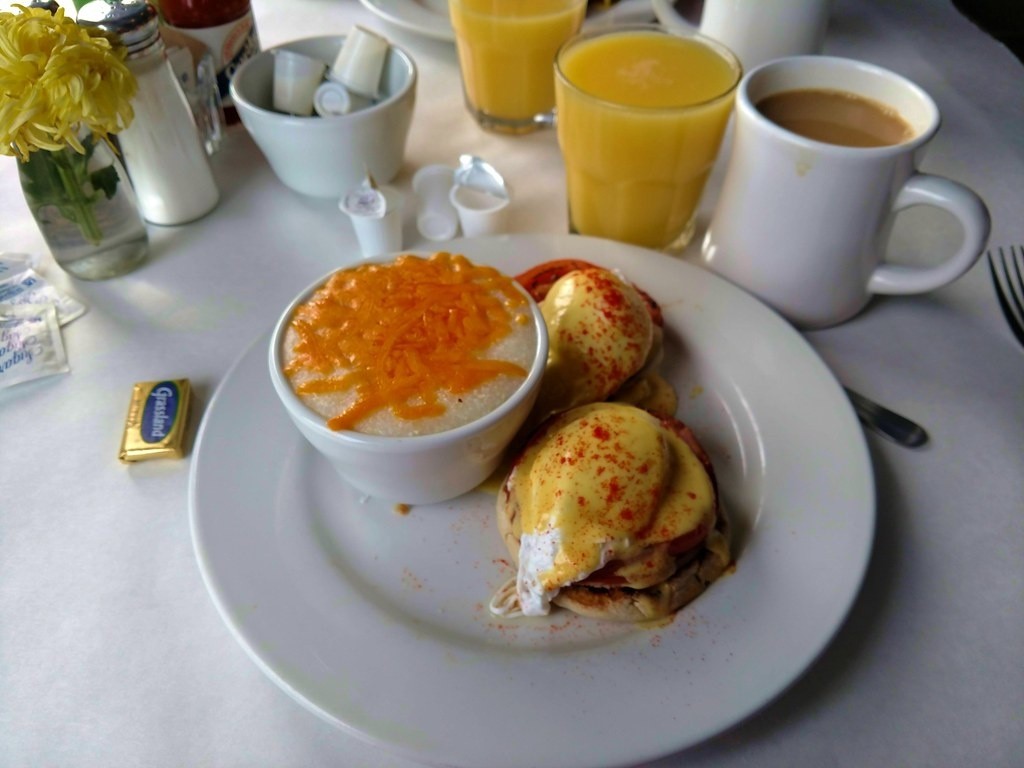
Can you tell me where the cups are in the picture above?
[271,24,390,118]
[702,56,990,329]
[553,22,743,255]
[343,168,509,256]
[651,0,831,75]
[449,0,588,135]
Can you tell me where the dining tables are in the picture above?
[0,0,1024,768]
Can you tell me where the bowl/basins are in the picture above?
[269,251,550,505]
[229,33,417,201]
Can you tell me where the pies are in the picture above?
[495,404,732,623]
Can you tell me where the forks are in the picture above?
[986,245,1024,351]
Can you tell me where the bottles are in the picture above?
[75,0,221,226]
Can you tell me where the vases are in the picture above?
[16,123,152,280]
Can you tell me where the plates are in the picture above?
[359,0,455,41]
[189,235,877,768]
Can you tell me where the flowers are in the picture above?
[0,4,139,247]
